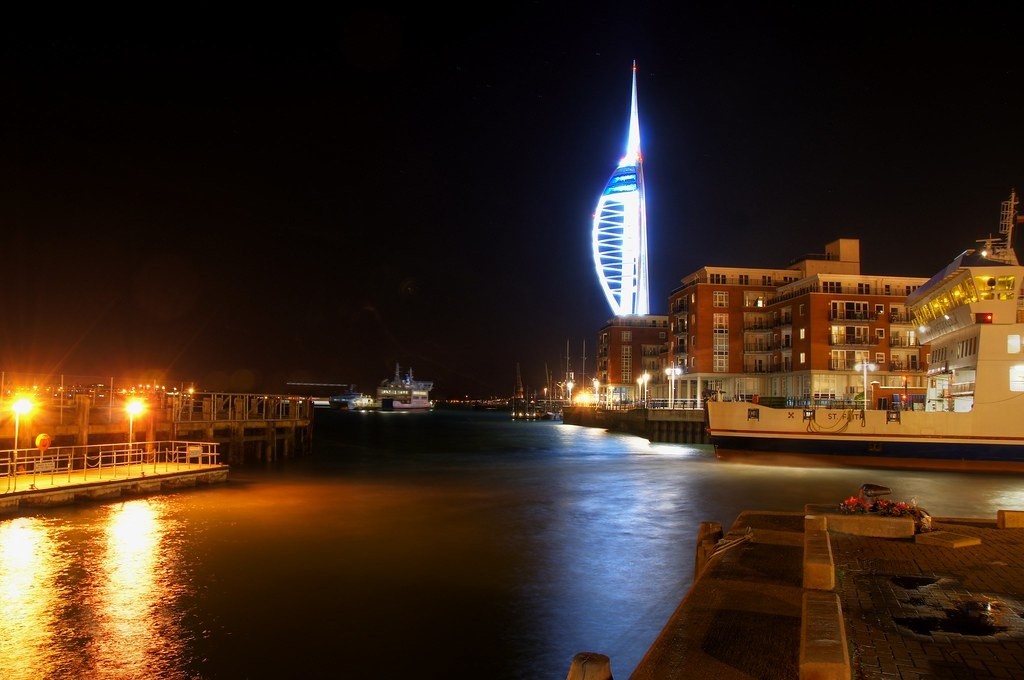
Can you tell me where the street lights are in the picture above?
[643,370,650,408]
[14,400,30,474]
[855,360,875,410]
[666,362,681,409]
[568,381,573,406]
[637,375,643,401]
[127,401,143,465]
[595,380,599,406]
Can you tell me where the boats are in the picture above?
[328,363,434,410]
[705,192,1024,476]
